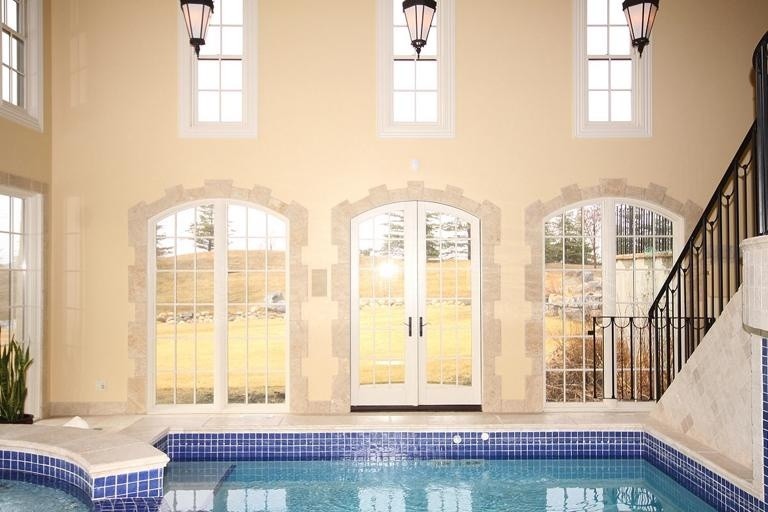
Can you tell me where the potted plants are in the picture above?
[1,335,40,427]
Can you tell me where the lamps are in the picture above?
[403,0,437,57]
[180,0,213,55]
[621,0,660,59]
